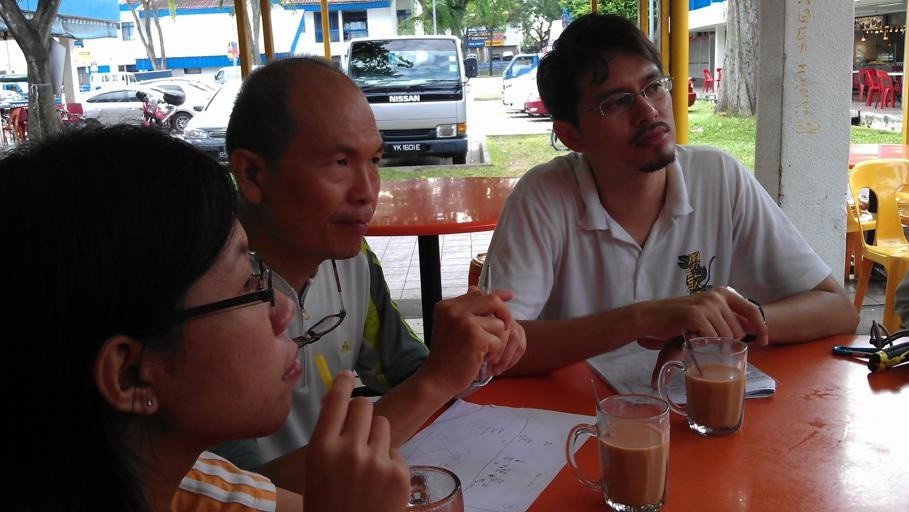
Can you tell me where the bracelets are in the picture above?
[741,293,768,345]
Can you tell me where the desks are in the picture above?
[235,330,908,512]
[358,174,521,355]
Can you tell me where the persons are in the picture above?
[0,127,411,511]
[472,11,863,378]
[206,57,528,491]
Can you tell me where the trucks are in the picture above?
[500,52,545,113]
[339,35,479,166]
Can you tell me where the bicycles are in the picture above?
[549,129,570,151]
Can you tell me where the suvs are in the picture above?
[502,66,541,108]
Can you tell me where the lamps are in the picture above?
[858,22,905,42]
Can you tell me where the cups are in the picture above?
[568,391,671,512]
[659,338,747,435]
[406,465,467,510]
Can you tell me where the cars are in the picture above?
[0,62,262,162]
[521,98,549,118]
[491,54,501,61]
[465,53,479,60]
[503,50,514,60]
[688,78,696,106]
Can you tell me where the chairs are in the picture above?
[852,65,904,110]
[848,156,909,331]
[702,69,715,102]
[714,65,723,88]
[1,96,89,151]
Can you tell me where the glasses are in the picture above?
[584,76,673,121]
[291,259,346,349]
[167,251,275,327]
